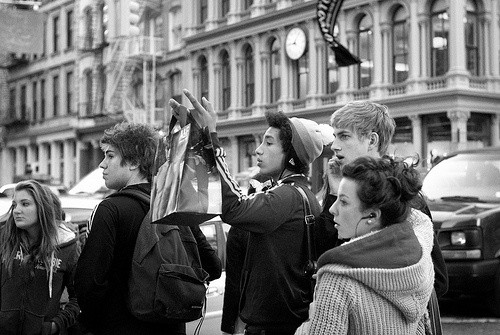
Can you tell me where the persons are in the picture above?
[294,157,436,335]
[73,121,223,335]
[318,101,449,335]
[0,180,81,335]
[169,88,323,335]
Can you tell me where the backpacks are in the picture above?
[107,189,211,325]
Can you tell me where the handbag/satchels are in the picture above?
[149,105,223,227]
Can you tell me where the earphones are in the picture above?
[361,213,376,219]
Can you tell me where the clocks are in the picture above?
[284,25,308,60]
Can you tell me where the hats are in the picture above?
[288,117,337,172]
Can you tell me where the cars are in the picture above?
[419,147,500,295]
[56,165,110,242]
[185,213,231,335]
